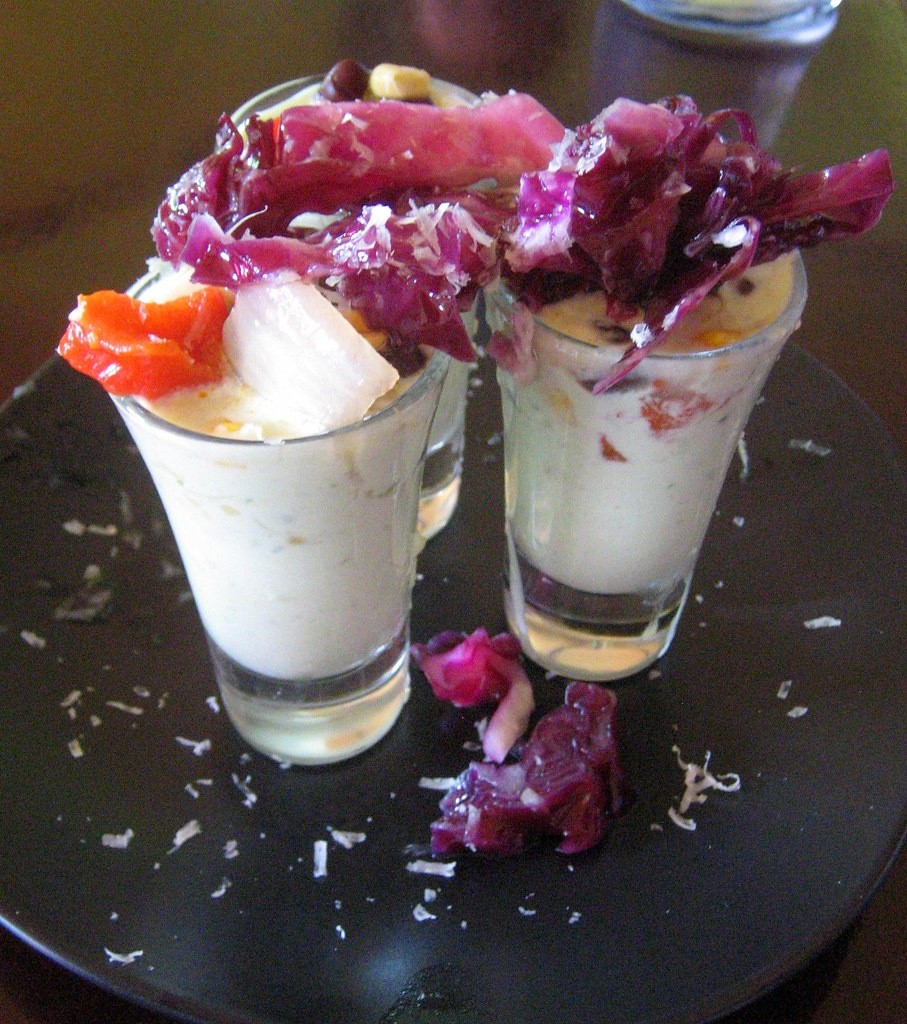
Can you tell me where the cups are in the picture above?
[216,73,489,541]
[478,247,809,682]
[103,272,465,771]
